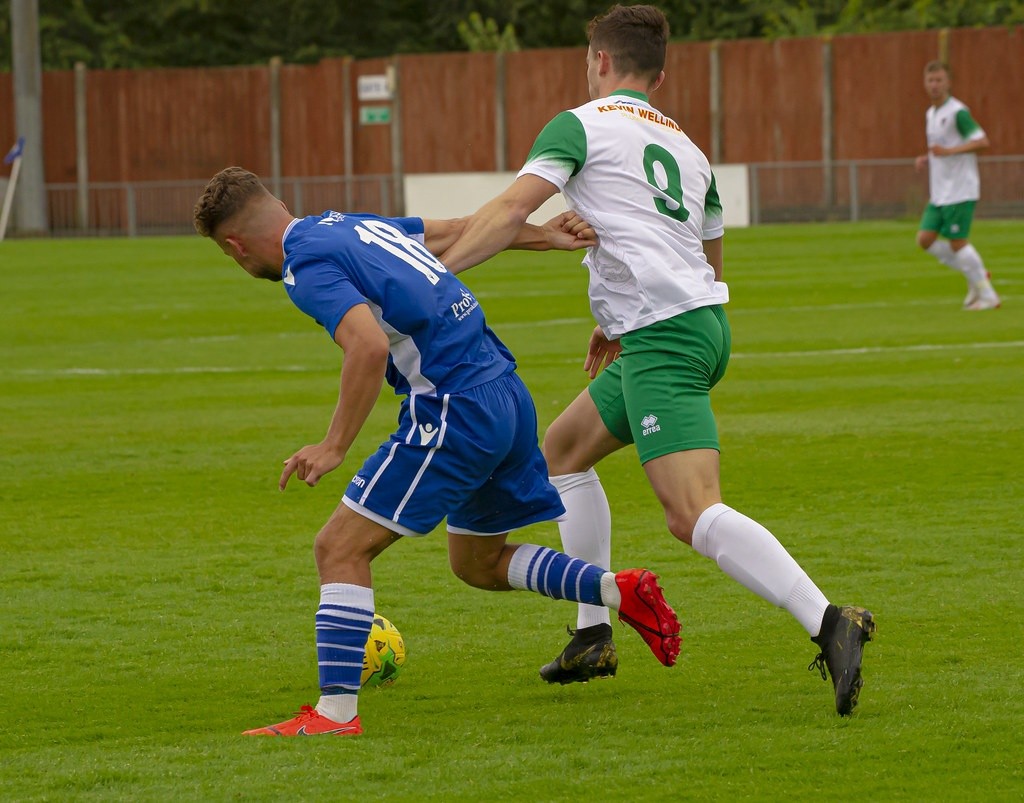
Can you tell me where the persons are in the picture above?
[196,166,681,733]
[433,4,875,714]
[914,60,1001,311]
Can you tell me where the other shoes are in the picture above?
[963,271,1001,311]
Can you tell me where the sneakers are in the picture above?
[814,603,876,717]
[615,568,682,666]
[538,639,619,685]
[241,711,363,738]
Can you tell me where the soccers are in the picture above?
[360,611,407,690]
